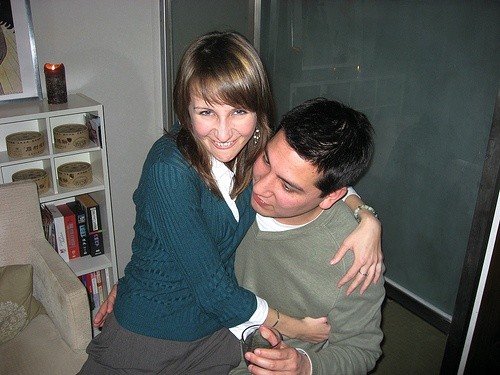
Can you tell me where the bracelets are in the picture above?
[354,205,378,224]
[272,309,279,327]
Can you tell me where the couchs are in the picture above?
[0,179,92,375]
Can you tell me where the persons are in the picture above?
[75,31,382,375]
[93,97,386,375]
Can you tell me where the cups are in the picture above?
[242,324,282,372]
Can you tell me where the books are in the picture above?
[41,194,104,263]
[78,267,114,321]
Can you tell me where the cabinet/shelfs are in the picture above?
[0,93,118,337]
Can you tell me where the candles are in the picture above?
[44,63,67,104]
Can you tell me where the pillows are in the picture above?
[0,264,45,345]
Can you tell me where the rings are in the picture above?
[359,271,368,276]
